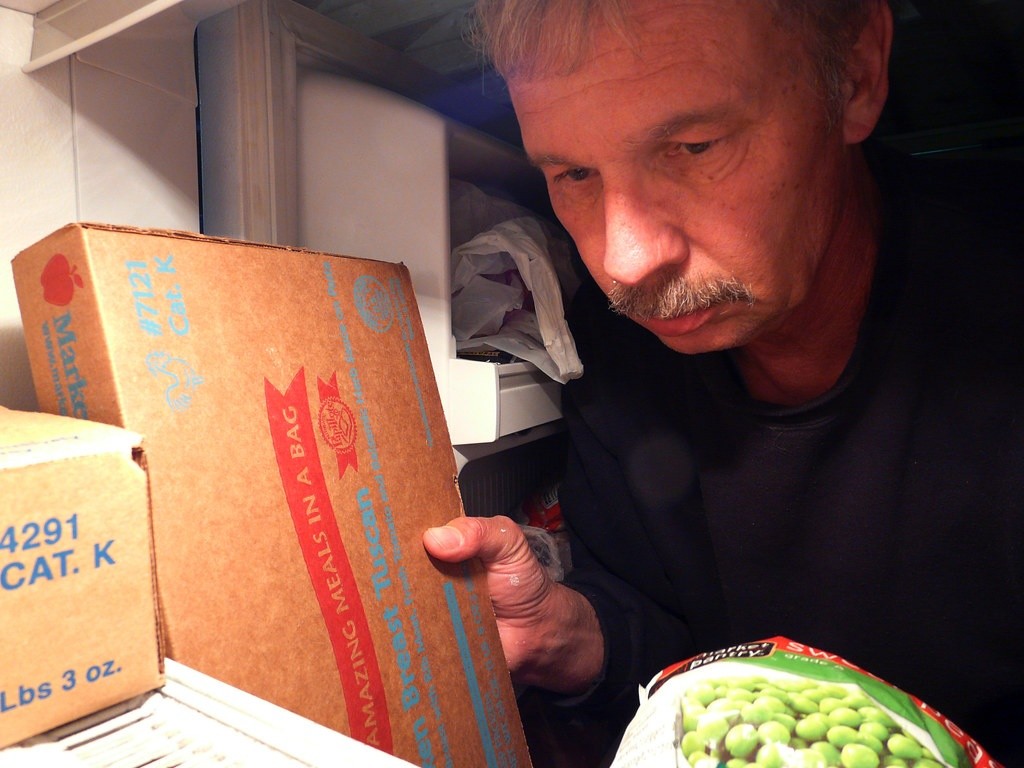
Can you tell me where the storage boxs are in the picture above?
[0,408,164,752]
[12,222,532,768]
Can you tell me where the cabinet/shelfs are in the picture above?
[195,1,564,494]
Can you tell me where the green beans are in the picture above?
[676,671,948,767]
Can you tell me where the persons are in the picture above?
[424,0,1024,768]
